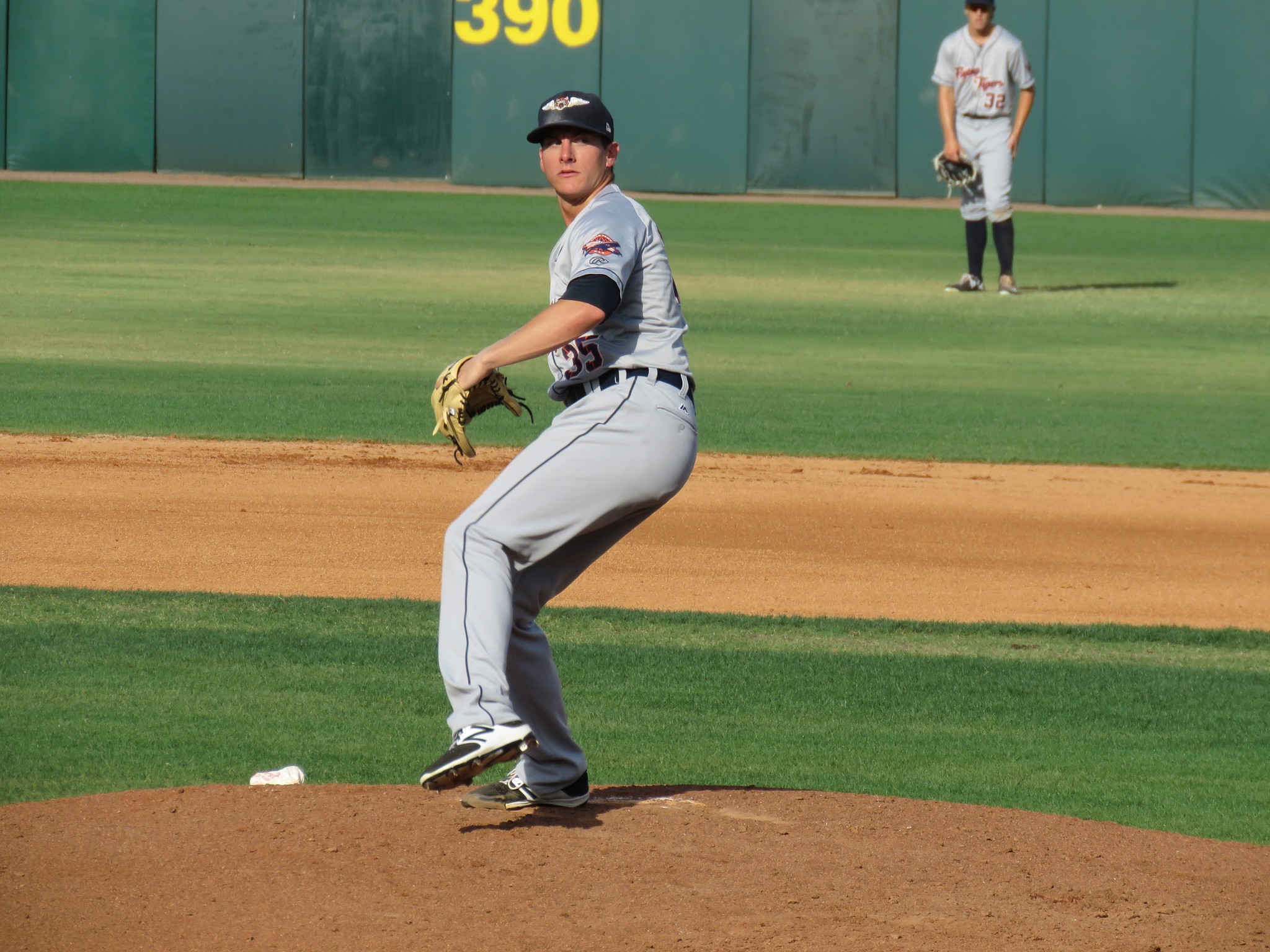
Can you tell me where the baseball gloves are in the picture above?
[430,355,523,458]
[933,151,977,187]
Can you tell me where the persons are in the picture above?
[419,91,700,812]
[931,0,1036,294]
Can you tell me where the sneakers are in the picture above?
[944,273,985,294]
[997,274,1018,297]
[420,720,537,798]
[460,766,591,811]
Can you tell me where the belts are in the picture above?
[963,113,1007,119]
[564,365,695,410]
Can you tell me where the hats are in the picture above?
[526,90,614,144]
